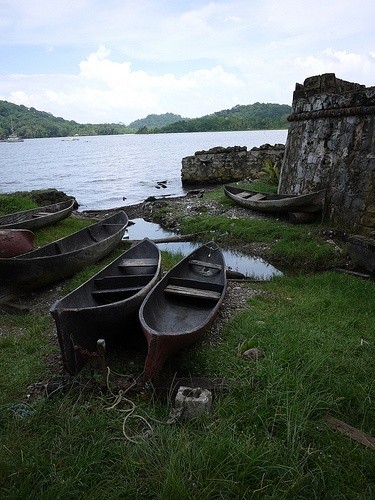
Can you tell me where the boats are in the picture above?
[7,117,24,143]
[223,185,328,214]
[0,198,75,231]
[138,240,227,388]
[50,236,161,378]
[0,209,129,318]
